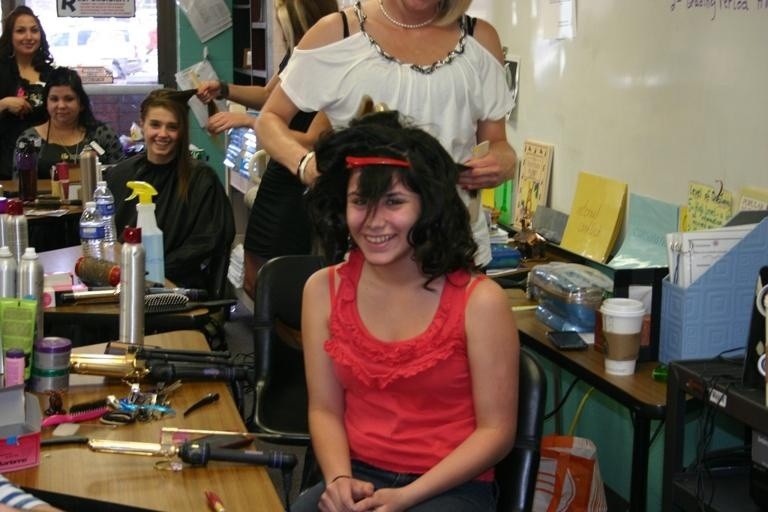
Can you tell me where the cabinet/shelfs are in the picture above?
[224,0,271,207]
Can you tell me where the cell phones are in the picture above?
[546,331,588,351]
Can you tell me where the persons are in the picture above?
[10,65,127,181]
[288,110,521,512]
[196,0,339,301]
[102,80,237,308]
[0,5,54,181]
[253,0,517,275]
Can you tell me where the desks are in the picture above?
[0,328,288,512]
[502,290,703,511]
[38,275,211,346]
[662,357,768,512]
[483,256,545,279]
[1,178,83,224]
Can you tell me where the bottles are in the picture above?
[78,201,105,260]
[5,199,29,265]
[73,255,123,287]
[27,365,70,395]
[118,227,144,345]
[0,196,10,250]
[2,348,26,387]
[31,335,73,369]
[13,138,100,208]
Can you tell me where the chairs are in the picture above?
[248,253,346,493]
[496,349,548,511]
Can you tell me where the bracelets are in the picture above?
[218,80,230,101]
[332,474,352,482]
[297,151,316,185]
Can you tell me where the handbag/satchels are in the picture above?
[531,433,608,512]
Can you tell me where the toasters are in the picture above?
[93,180,117,249]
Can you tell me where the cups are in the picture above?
[598,296,645,377]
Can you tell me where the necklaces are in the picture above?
[51,121,83,166]
[379,0,439,29]
